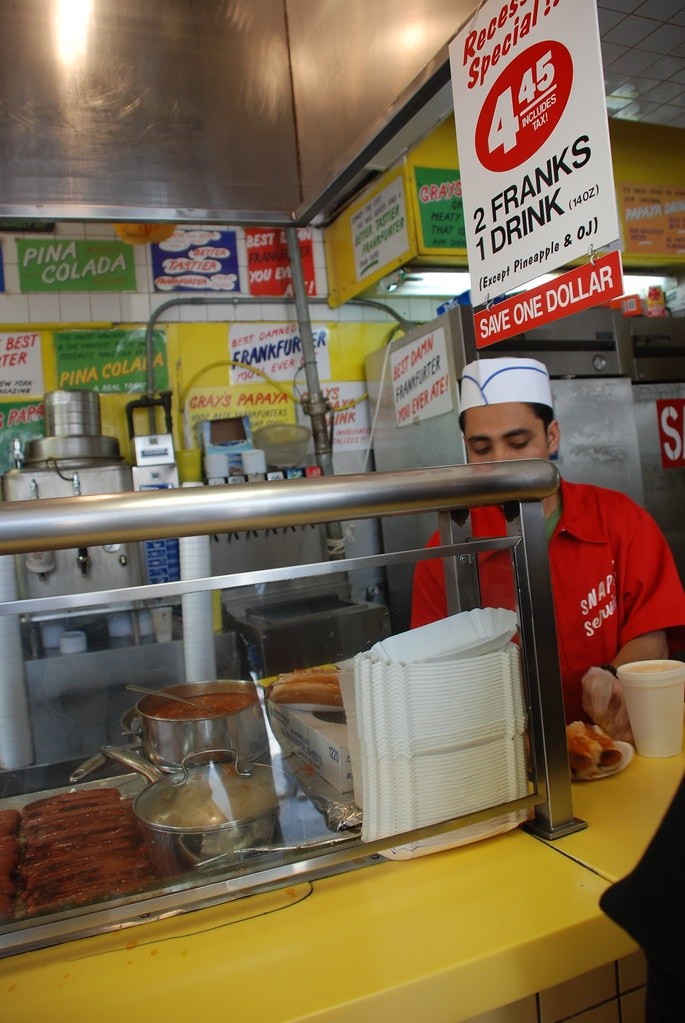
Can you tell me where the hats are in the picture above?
[458,357,554,437]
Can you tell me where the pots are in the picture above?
[100,744,296,879]
[119,679,270,774]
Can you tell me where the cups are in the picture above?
[150,606,173,643]
[616,660,685,758]
[176,448,202,483]
[59,630,88,656]
[647,285,665,318]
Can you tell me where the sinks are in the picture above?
[245,593,386,645]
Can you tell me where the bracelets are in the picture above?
[601,664,616,676]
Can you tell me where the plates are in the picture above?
[336,607,529,861]
[571,740,635,780]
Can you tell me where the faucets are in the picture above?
[76,547,90,577]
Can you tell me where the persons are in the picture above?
[414,357,685,745]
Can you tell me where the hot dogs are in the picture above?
[264,669,347,708]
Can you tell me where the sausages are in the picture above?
[565,724,593,771]
[0,788,160,923]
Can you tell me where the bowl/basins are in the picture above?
[252,423,311,469]
